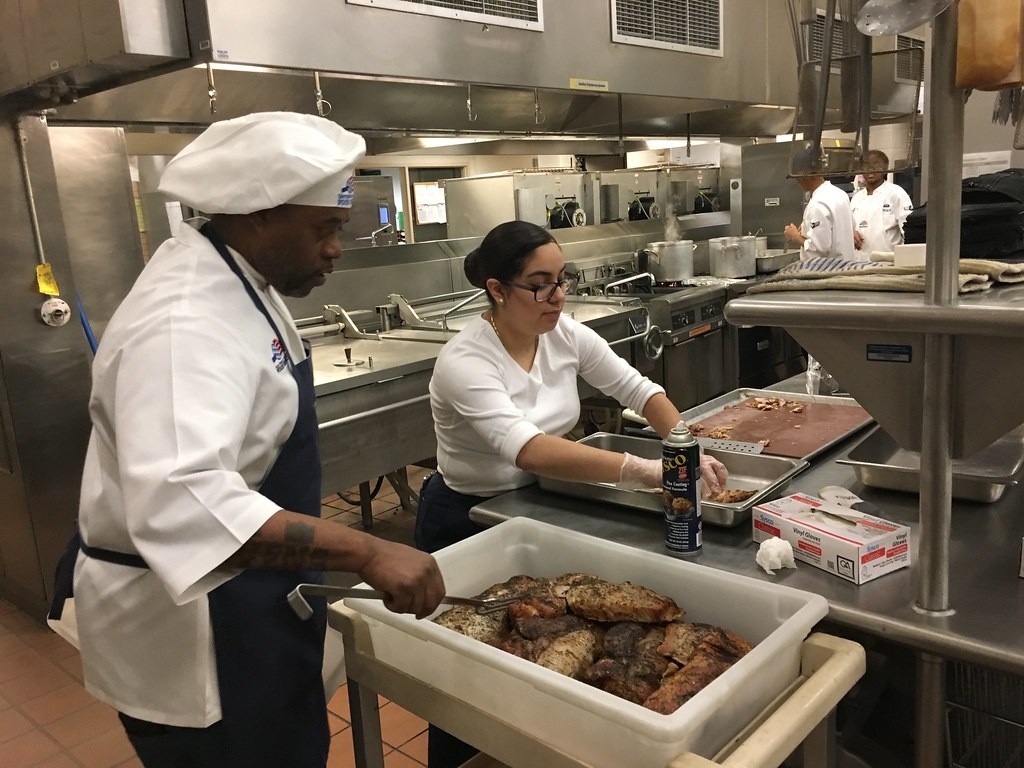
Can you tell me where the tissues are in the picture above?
[753,491,912,585]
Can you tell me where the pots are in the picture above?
[708,235,756,279]
[644,239,698,282]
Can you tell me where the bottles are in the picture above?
[662,420,703,558]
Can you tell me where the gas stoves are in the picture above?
[564,252,765,307]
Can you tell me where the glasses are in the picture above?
[502,271,581,303]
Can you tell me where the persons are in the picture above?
[784,145,855,263]
[850,150,914,261]
[413,220,727,554]
[44,110,446,768]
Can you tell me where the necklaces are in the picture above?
[490,311,505,346]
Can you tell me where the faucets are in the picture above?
[601,270,656,297]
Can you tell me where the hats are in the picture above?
[158,110,367,214]
